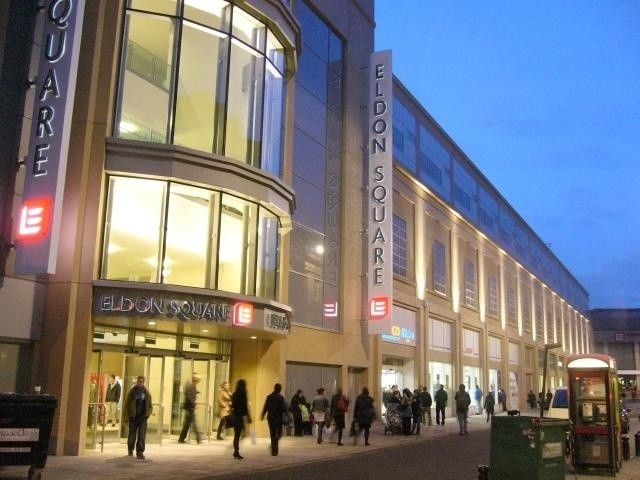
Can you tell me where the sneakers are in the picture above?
[127,448,145,460]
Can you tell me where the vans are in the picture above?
[548,387,570,420]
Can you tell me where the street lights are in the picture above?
[539,341,561,418]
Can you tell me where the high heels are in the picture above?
[233,451,245,460]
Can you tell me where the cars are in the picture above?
[620,399,631,432]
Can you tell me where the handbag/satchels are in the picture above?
[348,420,357,437]
[223,414,234,429]
[312,410,329,424]
[401,395,409,410]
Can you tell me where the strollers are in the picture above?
[384,402,403,436]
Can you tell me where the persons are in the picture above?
[288,383,506,445]
[261,383,289,456]
[537,392,542,408]
[527,390,537,409]
[546,389,553,402]
[125,376,153,459]
[216,381,232,440]
[104,374,121,427]
[231,378,251,460]
[177,374,204,444]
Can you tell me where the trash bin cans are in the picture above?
[490,416,573,480]
[0,392,58,480]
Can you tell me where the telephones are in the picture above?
[578,401,596,423]
